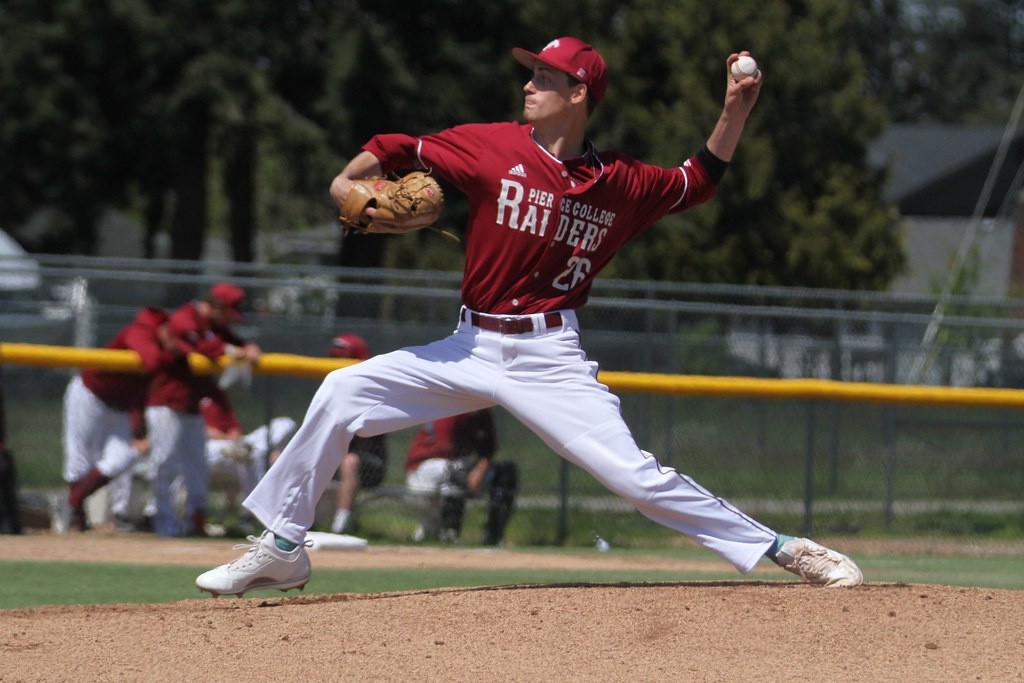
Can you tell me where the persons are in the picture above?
[269,334,388,536]
[195,36,862,599]
[43,281,296,538]
[404,408,519,546]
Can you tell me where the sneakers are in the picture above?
[770,536,863,587]
[196,529,312,599]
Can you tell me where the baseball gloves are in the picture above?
[336,170,446,236]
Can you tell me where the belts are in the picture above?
[462,308,563,335]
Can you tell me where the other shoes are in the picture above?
[47,491,72,535]
[332,510,359,533]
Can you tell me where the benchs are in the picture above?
[316,487,444,541]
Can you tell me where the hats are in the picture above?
[212,283,250,322]
[328,334,369,359]
[512,37,607,102]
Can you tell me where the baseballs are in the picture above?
[730,55,759,83]
